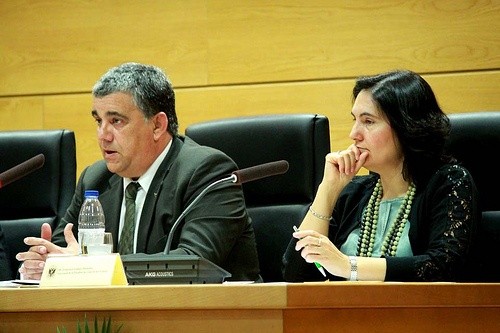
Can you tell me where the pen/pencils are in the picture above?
[293,226,326,277]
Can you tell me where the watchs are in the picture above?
[348,256,357,280]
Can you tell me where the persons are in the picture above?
[276,68,482,283]
[14,61,266,286]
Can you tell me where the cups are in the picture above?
[81,232,113,255]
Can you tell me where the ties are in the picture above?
[117,182,140,254]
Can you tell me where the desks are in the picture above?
[1,281,499,332]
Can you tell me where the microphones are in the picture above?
[119,160,289,285]
[0,154,45,187]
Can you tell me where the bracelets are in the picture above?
[309,206,333,221]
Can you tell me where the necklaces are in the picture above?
[355,176,418,259]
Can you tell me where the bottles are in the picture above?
[77,190,106,255]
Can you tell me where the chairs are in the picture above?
[184,114,330,283]
[444,111,500,283]
[0,129,77,282]
